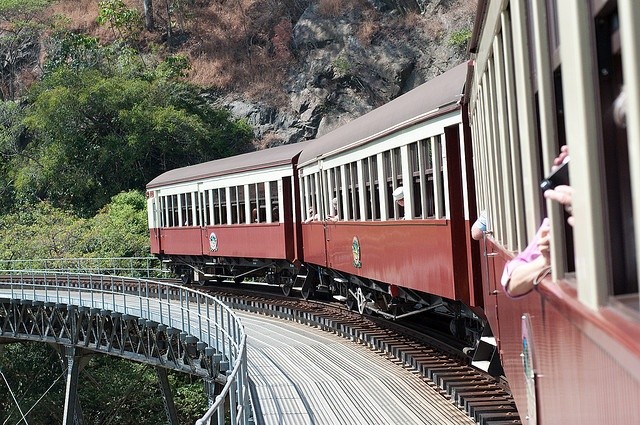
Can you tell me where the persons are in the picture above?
[305,207,320,224]
[273,206,279,220]
[542,145,575,228]
[394,187,405,208]
[471,211,488,241]
[328,198,338,222]
[501,217,555,300]
[252,209,257,221]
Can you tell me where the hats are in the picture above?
[393,187,404,200]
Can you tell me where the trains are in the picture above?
[144,1,639,425]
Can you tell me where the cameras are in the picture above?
[539,156,569,197]
